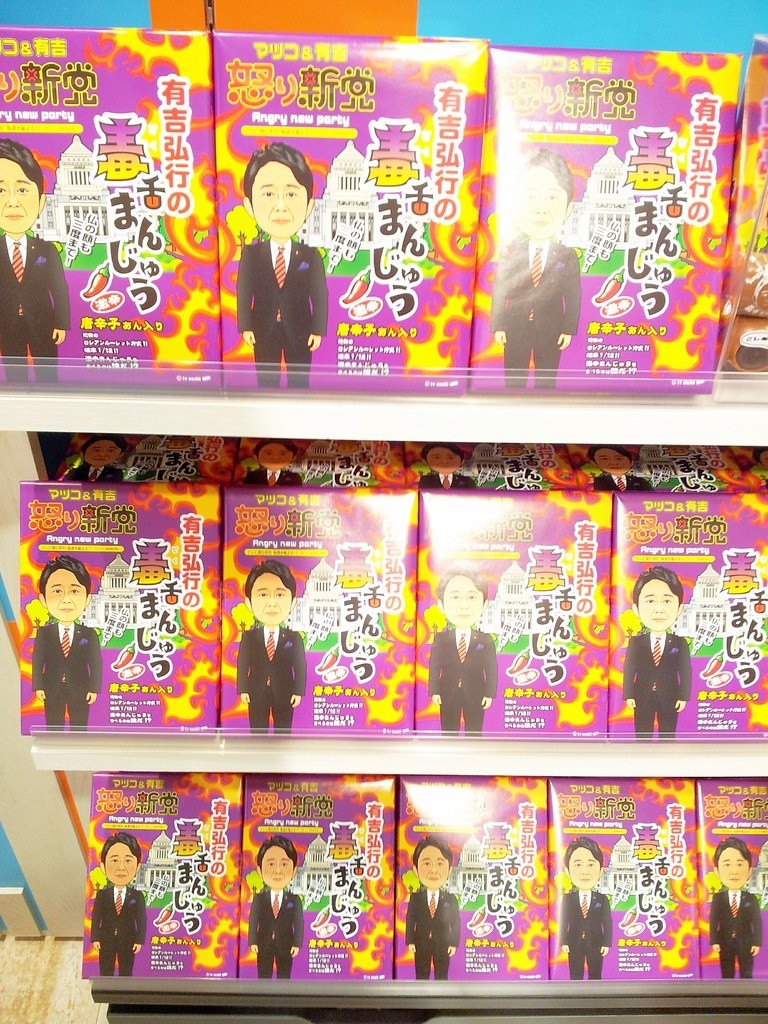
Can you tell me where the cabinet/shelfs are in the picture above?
[0,0,767,1023]
